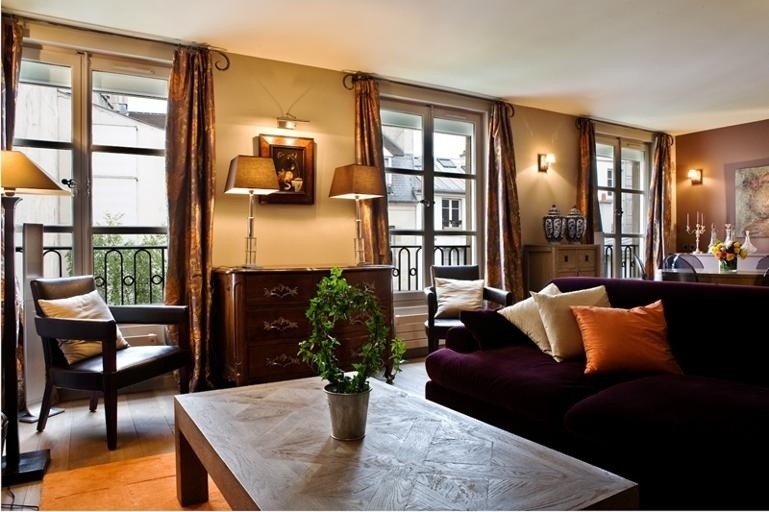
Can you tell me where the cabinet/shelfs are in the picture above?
[524,244,600,295]
[214,266,397,387]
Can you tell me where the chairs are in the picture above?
[424,265,512,353]
[31,276,190,451]
[632,254,769,286]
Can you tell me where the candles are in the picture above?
[687,210,704,226]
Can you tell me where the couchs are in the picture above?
[425,278,769,510]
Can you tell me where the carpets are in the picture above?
[40,452,231,510]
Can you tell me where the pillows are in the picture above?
[434,278,685,375]
[39,289,129,364]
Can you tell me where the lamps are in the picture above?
[275,117,311,129]
[329,164,386,267]
[539,153,556,171]
[2,152,73,485]
[224,154,281,268]
[688,169,703,185]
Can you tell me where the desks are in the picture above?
[173,372,638,510]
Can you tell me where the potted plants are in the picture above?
[298,269,406,442]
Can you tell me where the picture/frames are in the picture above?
[259,134,316,205]
[724,158,769,237]
